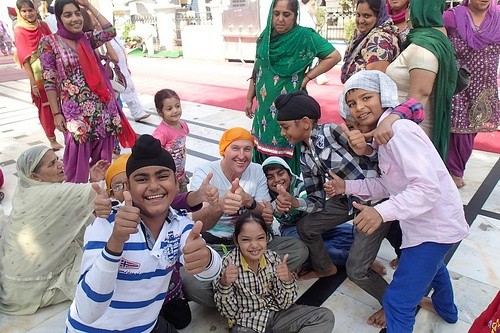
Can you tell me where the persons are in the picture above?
[65,135,222,333]
[92,154,220,330]
[0,146,111,315]
[179,128,309,307]
[323,70,470,333]
[53,112,61,116]
[274,92,390,329]
[152,89,190,193]
[0,0,500,268]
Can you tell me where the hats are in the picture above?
[106,154,131,193]
[274,91,321,122]
[126,134,177,178]
[219,126,254,156]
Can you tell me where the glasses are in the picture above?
[109,182,127,191]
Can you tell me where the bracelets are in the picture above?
[32,85,38,88]
[245,196,256,210]
[95,12,100,18]
